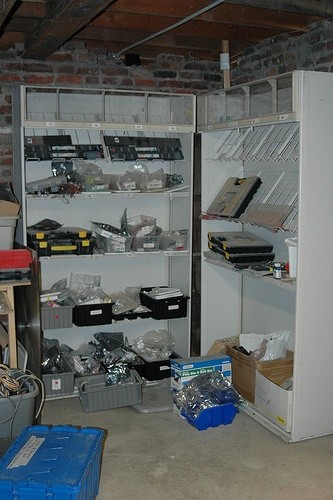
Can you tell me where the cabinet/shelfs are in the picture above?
[12,82,196,377]
[197,71,333,445]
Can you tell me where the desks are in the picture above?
[0,281,31,368]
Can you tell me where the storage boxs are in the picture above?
[254,365,292,432]
[0,199,21,250]
[206,336,240,356]
[0,368,40,437]
[28,157,188,257]
[226,339,293,405]
[173,389,183,419]
[129,344,182,381]
[102,347,146,378]
[76,372,143,412]
[73,301,115,327]
[0,424,108,500]
[171,354,232,390]
[42,355,76,395]
[139,285,190,320]
[41,297,75,329]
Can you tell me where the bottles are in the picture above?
[274,263,281,279]
